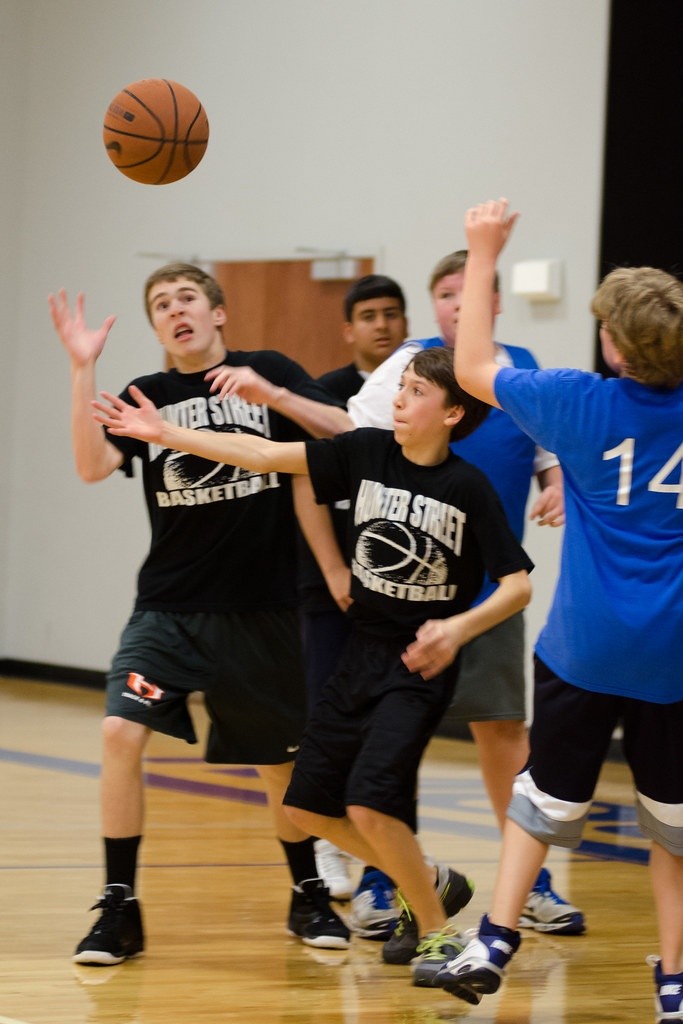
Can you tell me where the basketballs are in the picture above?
[103,76,210,186]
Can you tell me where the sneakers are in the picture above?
[517,868,586,933]
[383,864,475,963]
[434,913,522,1006]
[287,879,351,949]
[313,839,354,899]
[73,883,145,966]
[350,870,402,937]
[412,929,466,988]
[646,955,683,1024]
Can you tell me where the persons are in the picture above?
[48,264,352,967]
[293,248,589,940]
[314,274,407,402]
[433,195,683,1024]
[90,340,535,987]
